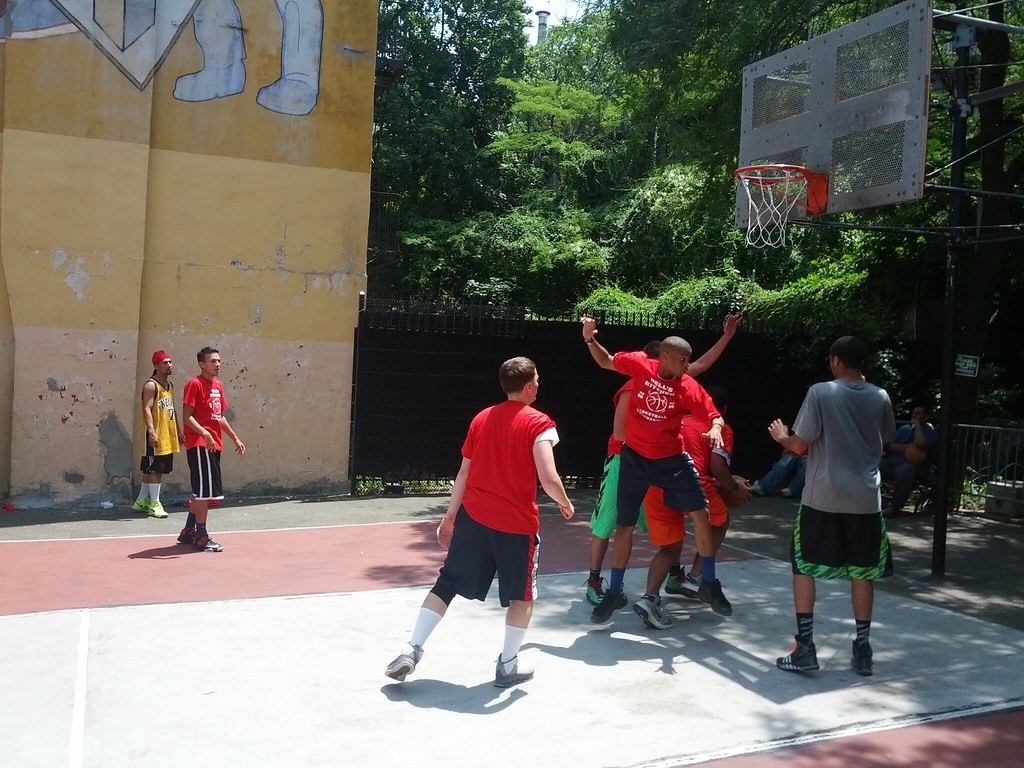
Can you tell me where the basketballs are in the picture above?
[718,476,750,505]
[905,445,928,466]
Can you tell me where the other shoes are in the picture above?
[781,488,794,498]
[882,506,898,518]
[749,480,762,497]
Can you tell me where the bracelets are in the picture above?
[584,337,596,346]
[713,423,723,429]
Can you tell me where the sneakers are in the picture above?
[698,578,732,616]
[495,653,534,688]
[591,588,627,624]
[132,499,151,512]
[634,594,673,629]
[385,641,424,682]
[148,502,169,518]
[177,529,196,544]
[665,566,703,598]
[777,635,819,670]
[851,639,874,676]
[192,532,224,552]
[586,577,609,606]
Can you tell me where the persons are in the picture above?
[381,356,575,689]
[878,406,940,519]
[581,314,732,625]
[766,336,896,676]
[586,314,743,606]
[747,445,810,499]
[178,348,246,552]
[633,410,751,630]
[132,350,185,517]
